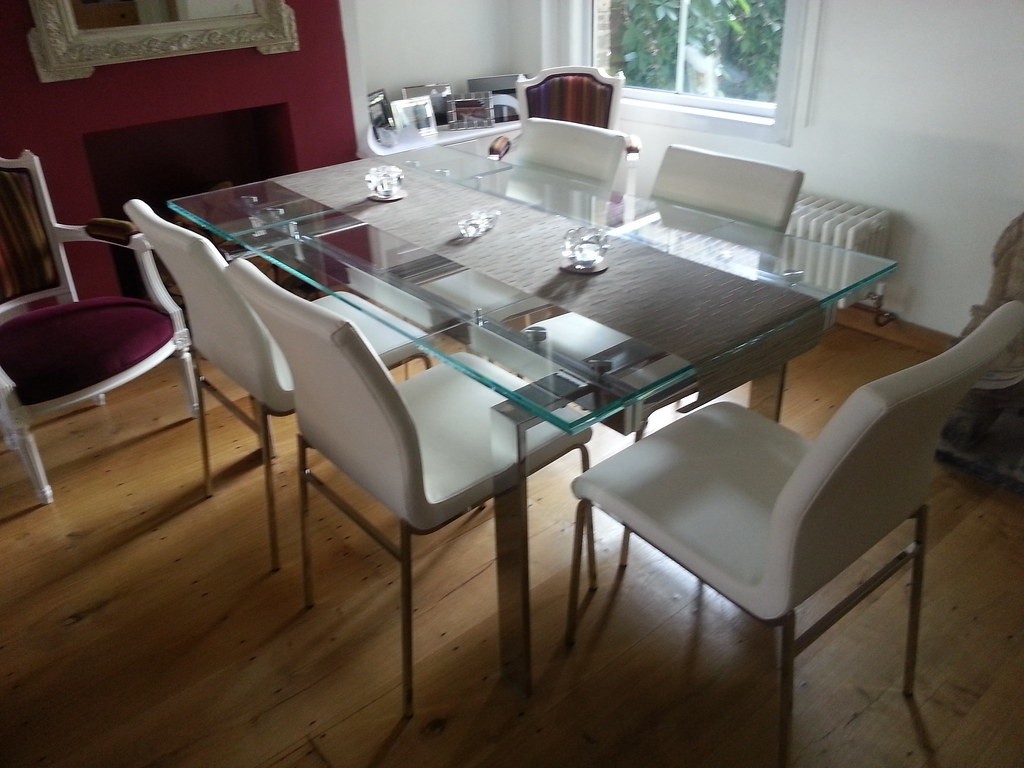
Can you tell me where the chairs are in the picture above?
[227,252,596,734]
[421,116,632,321]
[561,298,1024,768]
[514,65,626,131]
[634,141,806,442]
[125,197,438,577]
[0,149,199,509]
[935,209,1024,497]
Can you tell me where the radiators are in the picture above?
[775,194,900,333]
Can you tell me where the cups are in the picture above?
[563,227,611,270]
[366,165,405,197]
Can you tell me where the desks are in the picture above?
[167,147,900,689]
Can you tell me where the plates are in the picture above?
[365,191,406,200]
[560,258,609,274]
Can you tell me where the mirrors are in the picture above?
[26,0,302,85]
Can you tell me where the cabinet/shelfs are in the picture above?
[374,120,521,287]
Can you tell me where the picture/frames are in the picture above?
[366,89,397,143]
[401,82,457,126]
[389,95,439,139]
[446,91,496,133]
